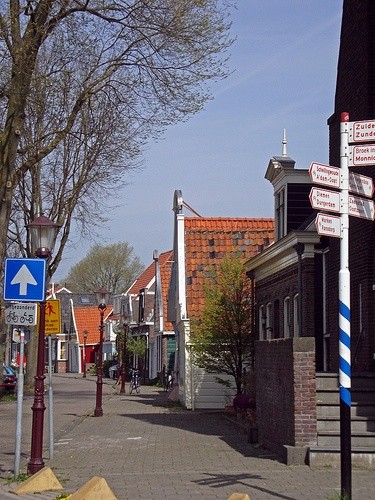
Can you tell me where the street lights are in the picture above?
[111,319,123,385]
[120,312,131,393]
[82,329,90,377]
[92,285,113,417]
[23,212,64,478]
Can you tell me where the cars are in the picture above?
[2,364,17,391]
